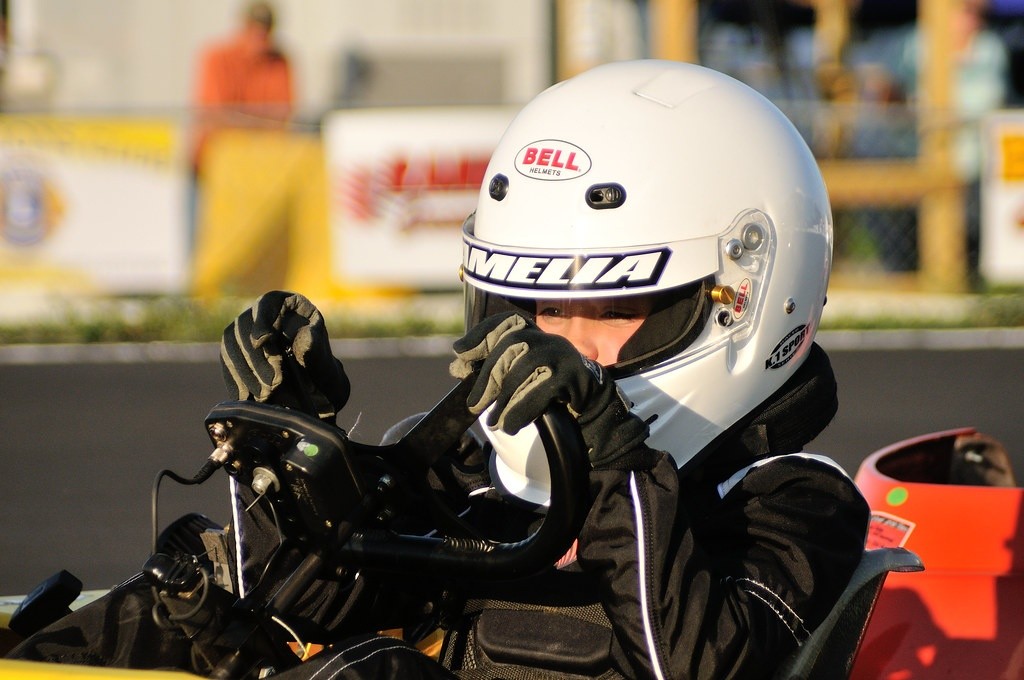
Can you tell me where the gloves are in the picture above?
[449,312,649,466]
[219,290,350,415]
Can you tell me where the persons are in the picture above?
[184,3,294,255]
[704,0,1007,268]
[4,61,869,679]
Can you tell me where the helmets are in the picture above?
[459,60,832,505]
